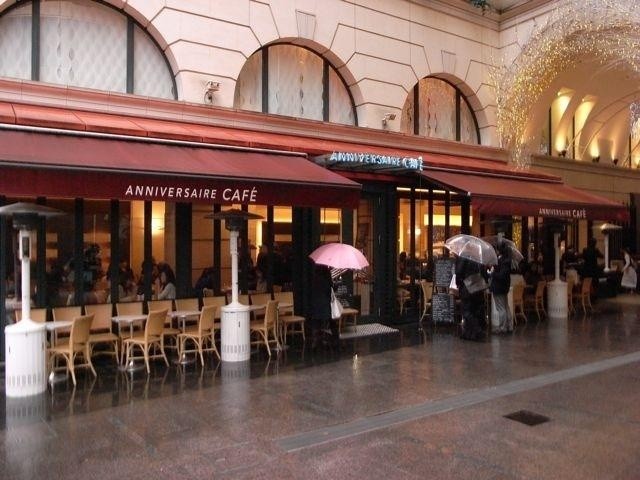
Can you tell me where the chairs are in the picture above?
[421,255,594,324]
[24,291,306,385]
[331,302,359,336]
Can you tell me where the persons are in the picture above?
[490,244,516,336]
[453,255,489,343]
[237,242,311,314]
[402,237,640,297]
[302,265,340,367]
[4,243,175,306]
[195,267,220,310]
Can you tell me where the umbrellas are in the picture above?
[308,239,370,270]
[444,232,499,267]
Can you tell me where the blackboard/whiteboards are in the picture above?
[431,260,456,324]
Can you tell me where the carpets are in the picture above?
[322,322,401,339]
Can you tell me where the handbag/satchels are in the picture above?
[463,273,493,294]
[331,287,344,320]
